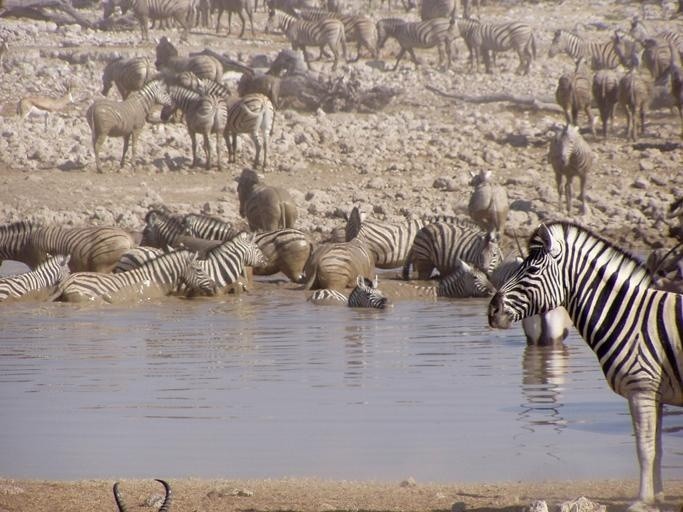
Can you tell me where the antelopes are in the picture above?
[14,80,76,134]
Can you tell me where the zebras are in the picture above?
[549,15,682,141]
[266,4,377,70]
[374,13,537,75]
[485,220,683,512]
[86,1,278,168]
[0,168,535,311]
[548,125,592,217]
